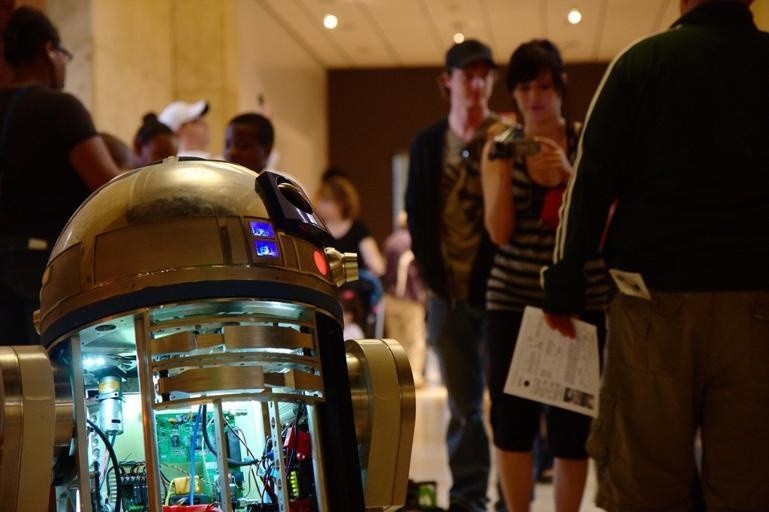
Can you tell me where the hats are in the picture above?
[158,100,208,133]
[447,40,498,68]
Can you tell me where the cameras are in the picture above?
[492,125,541,162]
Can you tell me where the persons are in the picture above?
[100,113,426,383]
[542,0,768,512]
[403,37,607,512]
[2,8,118,346]
